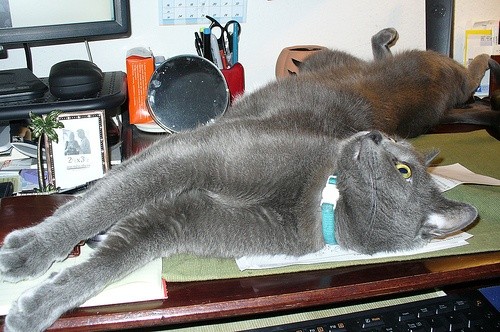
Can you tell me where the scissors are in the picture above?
[206,15,240,51]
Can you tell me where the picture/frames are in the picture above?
[42,110,110,190]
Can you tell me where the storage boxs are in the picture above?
[125,47,155,125]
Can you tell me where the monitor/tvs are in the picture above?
[0,0,131,58]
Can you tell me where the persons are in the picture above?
[62,129,90,155]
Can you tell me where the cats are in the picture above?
[0,27,500,332]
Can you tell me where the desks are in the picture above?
[0,122,500,332]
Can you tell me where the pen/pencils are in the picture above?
[204,28,213,62]
[200,27,205,40]
[233,22,238,64]
[194,31,204,56]
[220,50,227,70]
[223,31,233,68]
[211,33,224,70]
